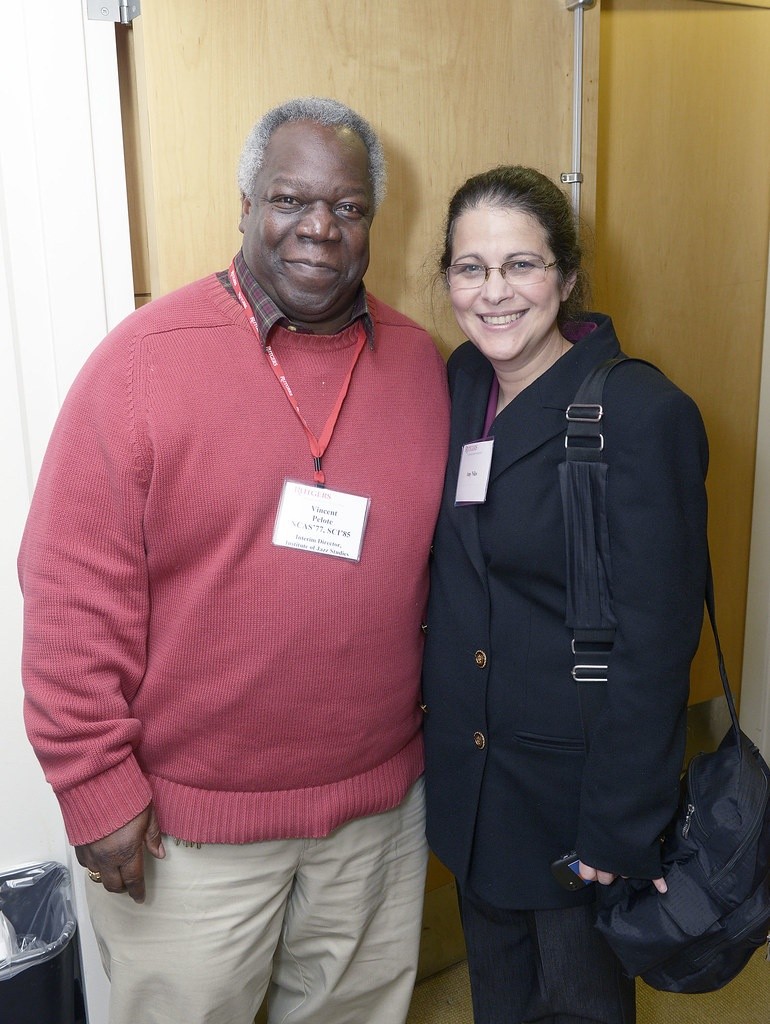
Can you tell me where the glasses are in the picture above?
[440,259,561,290]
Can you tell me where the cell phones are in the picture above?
[551,849,594,893]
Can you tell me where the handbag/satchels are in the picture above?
[590,724,770,994]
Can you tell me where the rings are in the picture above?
[87,869,101,880]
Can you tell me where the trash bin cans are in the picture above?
[0,858,82,1023]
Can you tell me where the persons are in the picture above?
[412,165,711,1024]
[17,97,450,1024]
